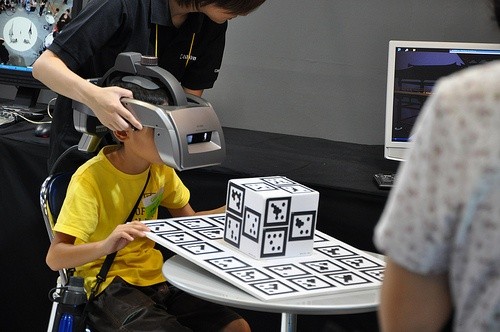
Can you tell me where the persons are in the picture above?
[46,126,251,332]
[372,0,500,332]
[32,0,266,187]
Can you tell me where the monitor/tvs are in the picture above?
[384,40,500,162]
[0,0,82,116]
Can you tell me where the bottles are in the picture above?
[49,275,86,332]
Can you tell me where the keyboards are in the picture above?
[373,174,394,189]
[0,107,26,127]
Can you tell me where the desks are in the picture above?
[162,251,387,332]
[0,98,402,332]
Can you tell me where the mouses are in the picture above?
[35,123,52,138]
